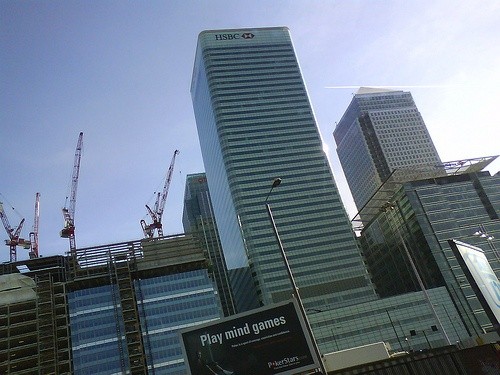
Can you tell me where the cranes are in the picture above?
[29,193,40,259]
[60,132,84,251]
[140,150,180,239]
[0,193,30,262]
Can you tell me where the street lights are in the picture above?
[264,178,331,375]
[473,223,500,263]
[379,201,454,347]
